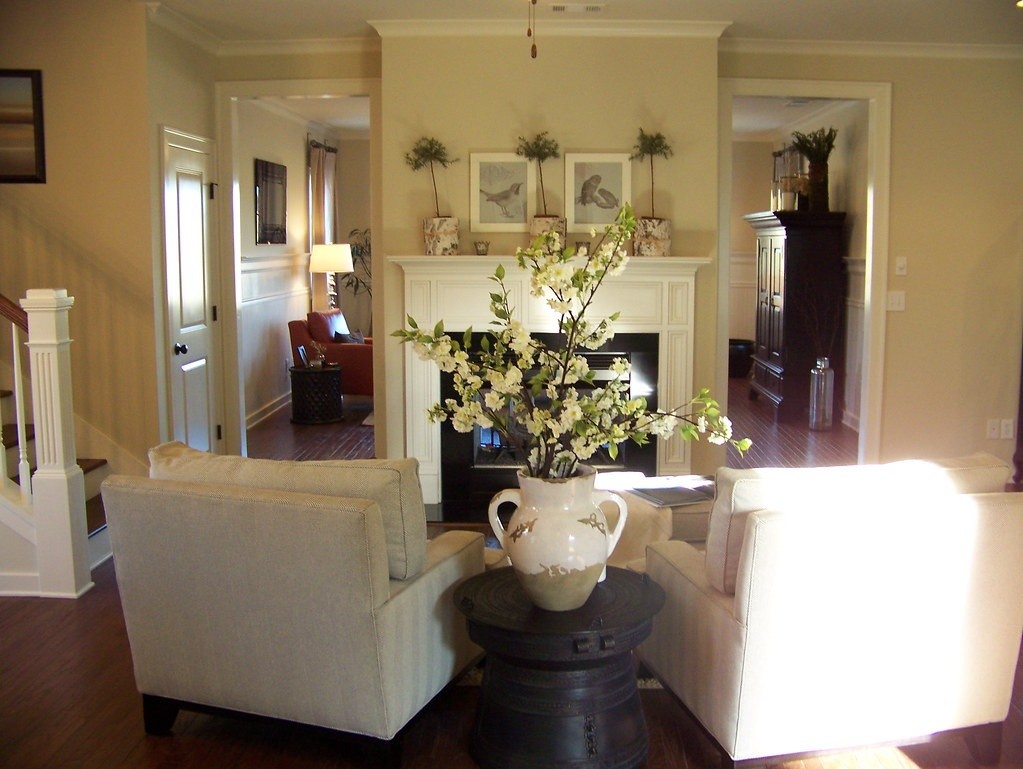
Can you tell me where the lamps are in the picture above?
[310,244,354,309]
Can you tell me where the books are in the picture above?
[623,474,715,508]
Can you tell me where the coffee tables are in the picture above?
[595,473,715,542]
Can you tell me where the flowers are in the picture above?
[391,202,758,478]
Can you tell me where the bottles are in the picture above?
[809,357,834,432]
[771,174,809,211]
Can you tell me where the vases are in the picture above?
[488,468,629,614]
[810,357,833,431]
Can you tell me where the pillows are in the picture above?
[334,328,365,343]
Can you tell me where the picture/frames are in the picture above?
[562,153,633,236]
[469,153,536,233]
[0,69,47,185]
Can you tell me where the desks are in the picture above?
[290,365,344,425]
[456,567,664,769]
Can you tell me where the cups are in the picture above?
[474,240,490,254]
[576,241,590,256]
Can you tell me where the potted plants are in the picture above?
[629,129,672,256]
[517,131,567,254]
[793,129,837,212]
[407,140,463,256]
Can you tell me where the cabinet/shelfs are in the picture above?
[742,209,853,410]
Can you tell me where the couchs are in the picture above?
[100,439,505,745]
[289,309,373,395]
[635,458,1023,759]
